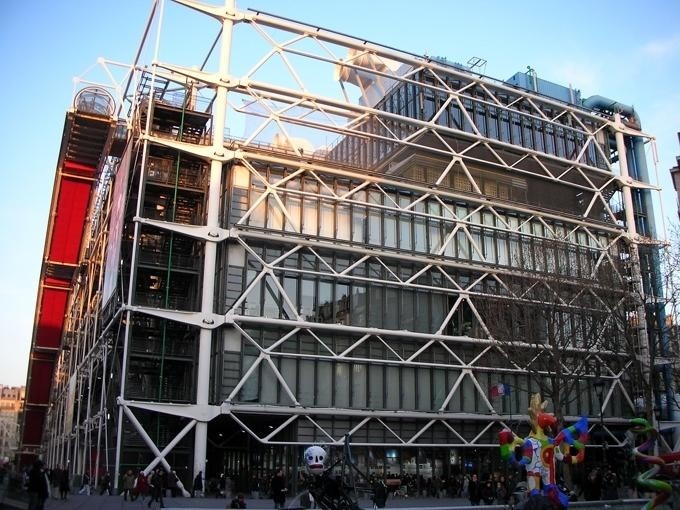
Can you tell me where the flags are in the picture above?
[490,384,510,398]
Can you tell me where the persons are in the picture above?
[75,467,204,510]
[366,469,531,509]
[558,467,657,504]
[1,456,72,510]
[209,468,309,510]
